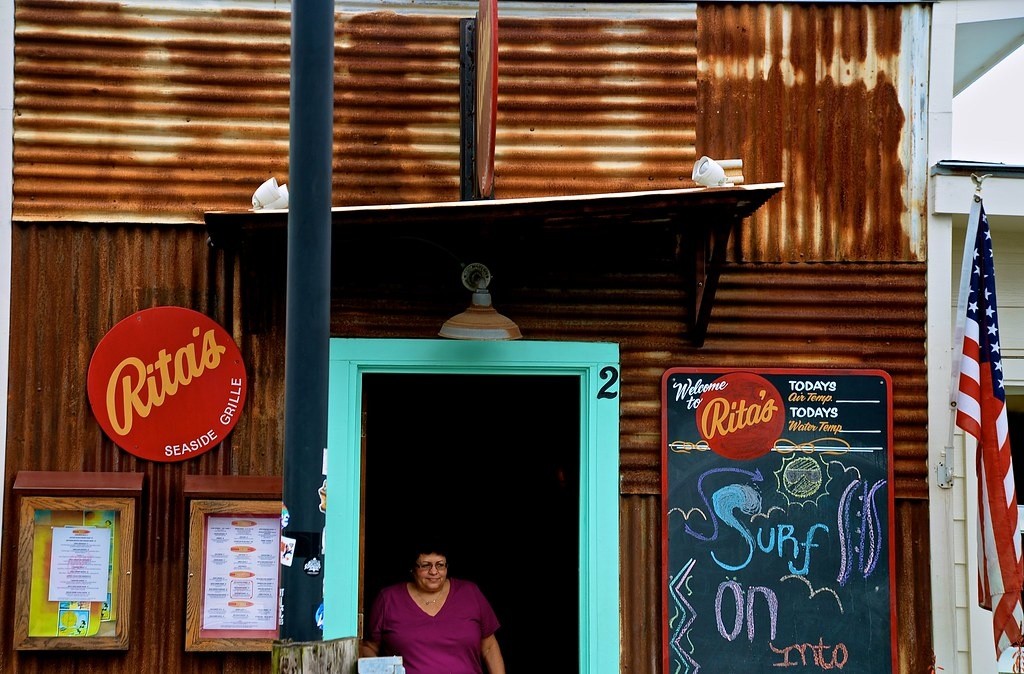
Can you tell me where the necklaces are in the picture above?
[416,588,439,605]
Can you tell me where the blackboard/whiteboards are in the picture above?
[660,365,903,673]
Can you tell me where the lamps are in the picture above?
[692,155,736,188]
[440,263,523,341]
[252,175,290,211]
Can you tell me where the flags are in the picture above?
[952,200,1024,674]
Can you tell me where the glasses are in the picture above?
[416,559,448,571]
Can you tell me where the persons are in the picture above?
[363,537,505,674]
